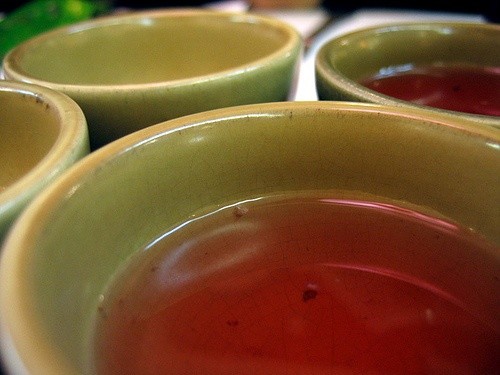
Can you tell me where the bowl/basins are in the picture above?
[0,0,499,374]
